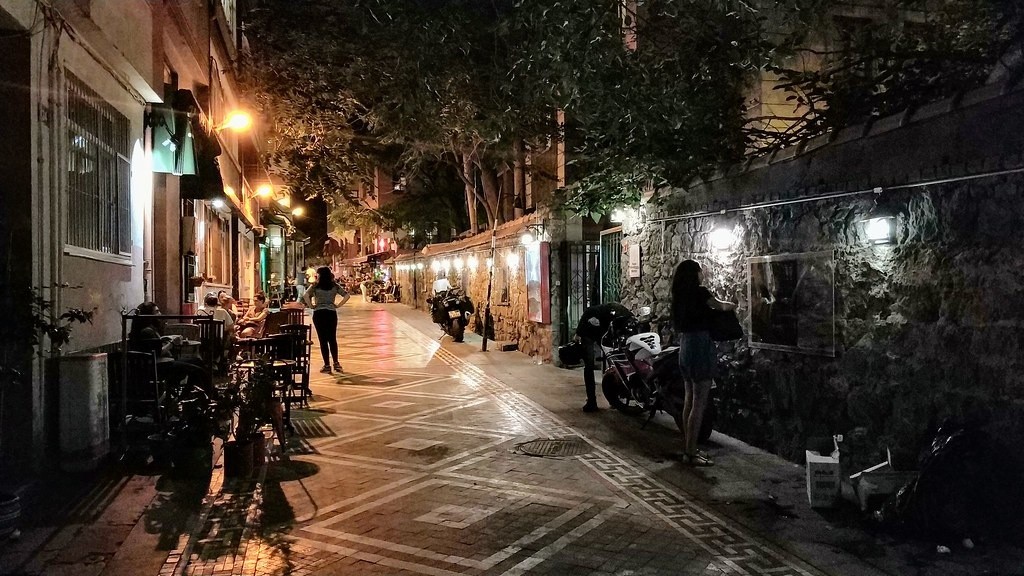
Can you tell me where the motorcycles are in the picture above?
[423,278,475,343]
[599,305,719,444]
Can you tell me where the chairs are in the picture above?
[165,299,314,449]
[117,346,179,443]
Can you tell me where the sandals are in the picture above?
[684,448,712,459]
[681,452,715,467]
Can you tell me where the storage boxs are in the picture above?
[805,435,841,509]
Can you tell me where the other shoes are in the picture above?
[320,365,331,374]
[583,401,597,412]
[333,362,342,369]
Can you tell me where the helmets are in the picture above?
[559,342,581,365]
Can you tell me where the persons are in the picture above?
[189,288,268,379]
[576,302,651,412]
[269,260,398,308]
[303,267,350,374]
[669,260,744,467]
[128,302,224,402]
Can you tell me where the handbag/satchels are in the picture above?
[709,308,743,342]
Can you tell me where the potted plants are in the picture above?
[215,353,271,485]
[0,272,100,540]
[247,359,278,479]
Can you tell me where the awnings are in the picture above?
[153,83,226,203]
